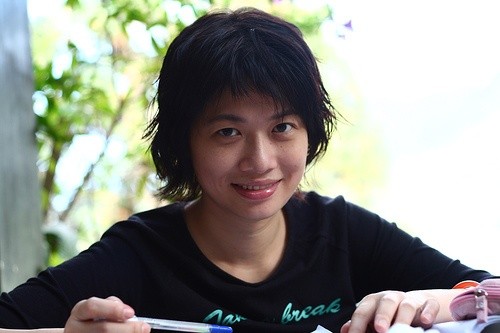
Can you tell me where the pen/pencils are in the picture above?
[125,314,237,332]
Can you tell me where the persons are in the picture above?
[0,8,500,333]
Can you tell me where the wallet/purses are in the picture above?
[449,277,500,324]
